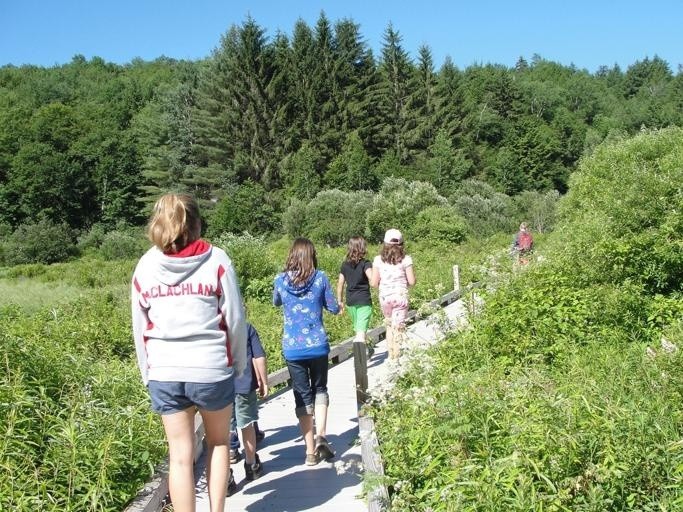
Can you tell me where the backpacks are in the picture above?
[520,232,531,249]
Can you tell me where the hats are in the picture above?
[384,229,403,244]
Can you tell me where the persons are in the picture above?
[273,237,341,466]
[336,227,416,365]
[511,222,534,272]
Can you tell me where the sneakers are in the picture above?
[230,449,242,464]
[226,468,243,497]
[244,453,263,480]
[256,429,265,442]
[305,435,336,466]
[367,348,375,360]
